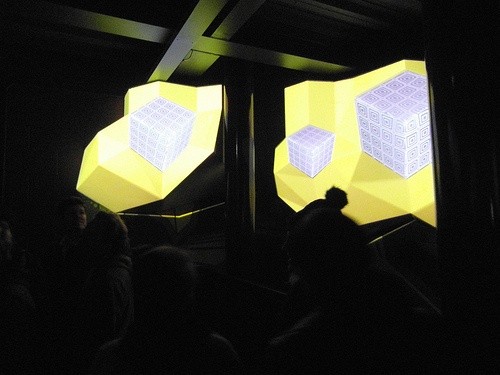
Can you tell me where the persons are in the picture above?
[92,246,243,375]
[0,223,29,302]
[67,213,135,375]
[265,208,403,374]
[42,197,88,274]
[281,187,346,294]
